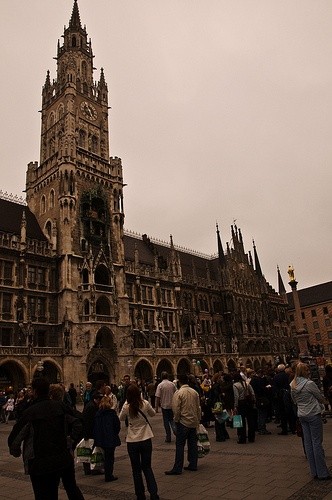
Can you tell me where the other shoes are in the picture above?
[164,469,182,475]
[277,432,288,435]
[150,494,160,500]
[105,475,118,482]
[184,466,197,471]
[136,495,146,500]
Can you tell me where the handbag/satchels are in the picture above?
[89,446,104,470]
[233,414,243,428]
[75,438,94,463]
[211,401,223,414]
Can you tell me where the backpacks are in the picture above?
[240,380,254,405]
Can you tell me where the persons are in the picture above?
[8,376,63,500]
[49,383,87,500]
[119,385,159,500]
[290,362,332,480]
[0,354,332,477]
